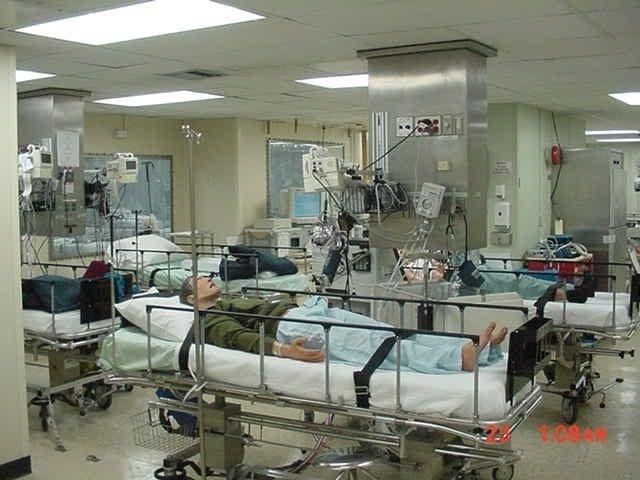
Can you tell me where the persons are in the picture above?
[401,259,596,304]
[178,274,510,373]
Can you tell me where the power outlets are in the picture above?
[399,116,441,138]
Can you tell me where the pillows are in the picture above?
[107,234,184,267]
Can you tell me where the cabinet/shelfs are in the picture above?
[246,224,318,257]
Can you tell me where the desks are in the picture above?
[172,232,215,249]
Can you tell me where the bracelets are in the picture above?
[273,342,286,358]
